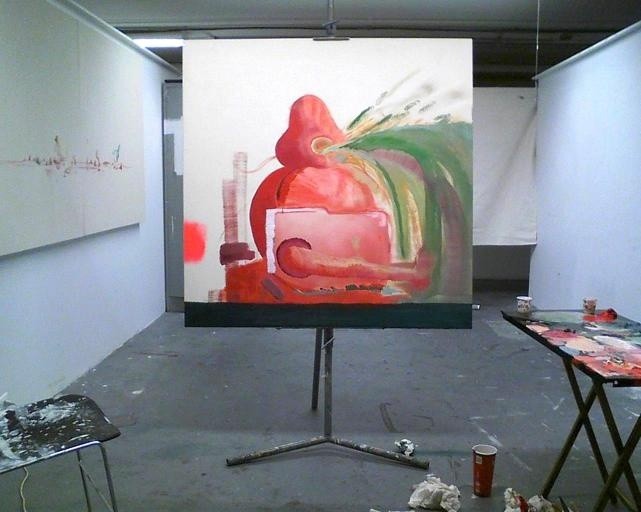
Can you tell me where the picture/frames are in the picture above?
[181,35,476,329]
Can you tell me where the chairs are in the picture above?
[2,393,120,512]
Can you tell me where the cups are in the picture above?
[472,443,499,497]
[517,296,534,314]
[583,298,599,314]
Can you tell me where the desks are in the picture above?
[501,308,641,512]
